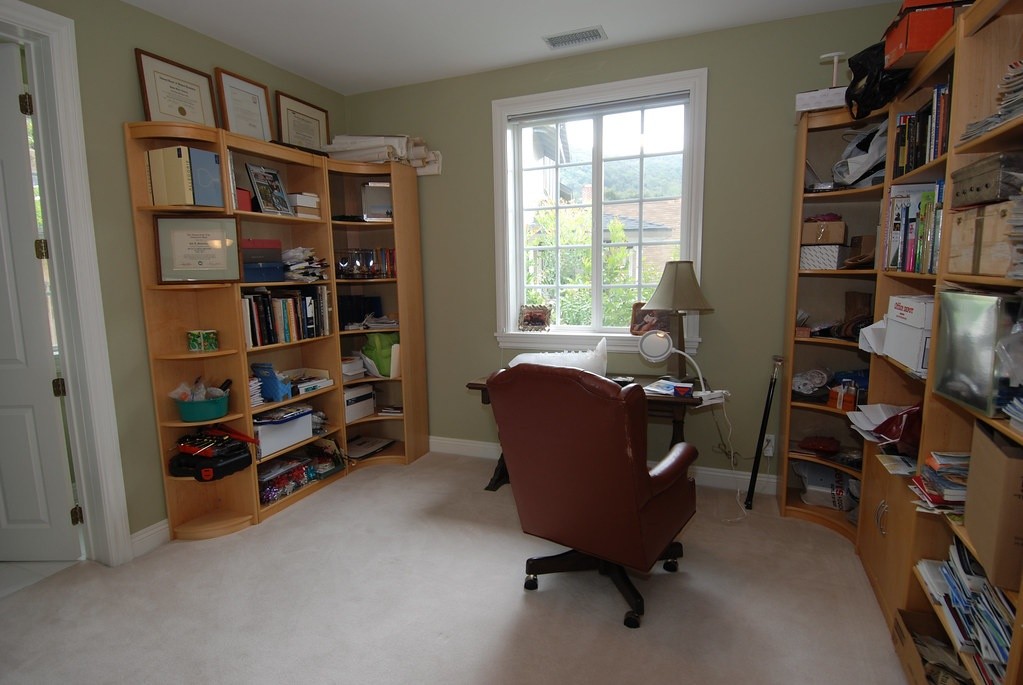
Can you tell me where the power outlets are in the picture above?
[763,434,776,456]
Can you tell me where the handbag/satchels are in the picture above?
[833,120,890,184]
[845,40,912,119]
[363,333,398,376]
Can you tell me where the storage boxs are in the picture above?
[254,411,312,458]
[963,418,1022,592]
[795,85,849,112]
[946,207,982,276]
[800,245,848,270]
[879,1,954,69]
[887,294,933,329]
[979,200,1016,277]
[883,315,934,373]
[801,220,847,248]
[344,383,374,422]
[851,236,876,256]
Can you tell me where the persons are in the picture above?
[633,309,657,331]
[524,312,544,326]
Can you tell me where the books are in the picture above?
[907,450,971,524]
[241,286,333,348]
[954,60,1023,147]
[1002,397,1023,433]
[882,71,954,275]
[915,537,1016,685]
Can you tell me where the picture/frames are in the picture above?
[519,305,552,331]
[630,302,671,337]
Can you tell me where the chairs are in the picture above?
[485,364,699,628]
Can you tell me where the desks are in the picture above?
[466,371,702,492]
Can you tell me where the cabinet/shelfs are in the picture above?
[776,0,1023,685]
[124,119,429,542]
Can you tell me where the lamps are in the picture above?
[640,261,714,380]
[638,330,725,409]
